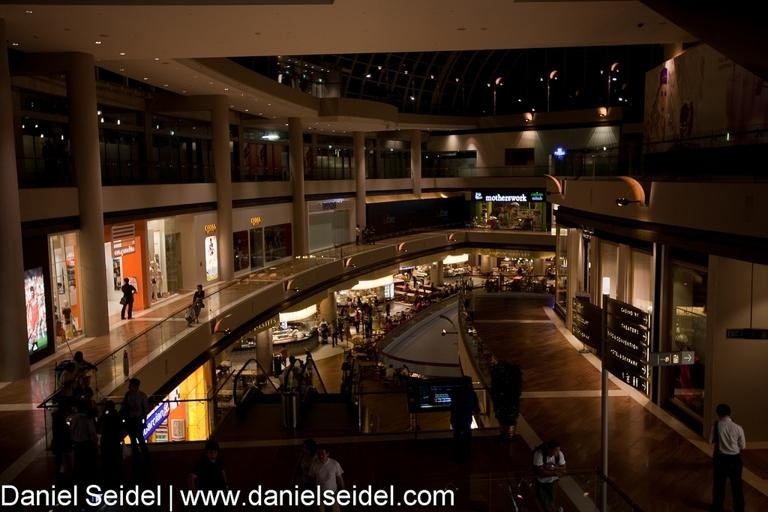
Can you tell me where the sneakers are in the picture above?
[121,316,135,319]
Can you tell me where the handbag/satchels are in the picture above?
[120,297,127,305]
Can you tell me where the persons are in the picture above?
[45,349,123,511]
[447,374,482,431]
[262,249,569,395]
[355,224,361,245]
[533,437,569,511]
[185,303,196,325]
[646,67,675,140]
[709,402,749,512]
[192,284,206,318]
[368,225,377,245]
[120,377,150,448]
[191,437,231,511]
[119,276,136,320]
[294,438,318,486]
[308,445,347,511]
